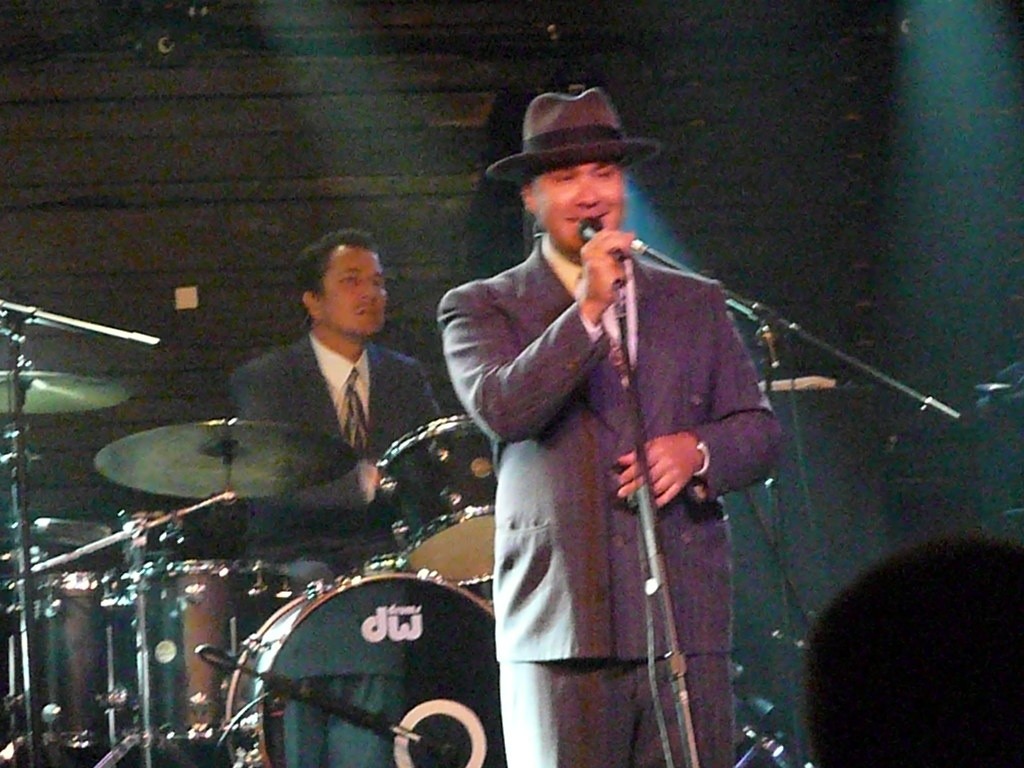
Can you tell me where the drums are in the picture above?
[0,568,123,750]
[224,572,508,768]
[123,560,295,740]
[377,414,506,585]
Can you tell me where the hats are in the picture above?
[484,88,664,183]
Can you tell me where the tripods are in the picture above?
[0,298,207,768]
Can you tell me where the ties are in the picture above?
[344,368,370,454]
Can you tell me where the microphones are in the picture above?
[417,739,461,768]
[578,215,625,301]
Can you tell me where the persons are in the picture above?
[228,230,442,487]
[434,86,788,768]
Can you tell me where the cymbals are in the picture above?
[93,419,358,500]
[0,369,131,415]
